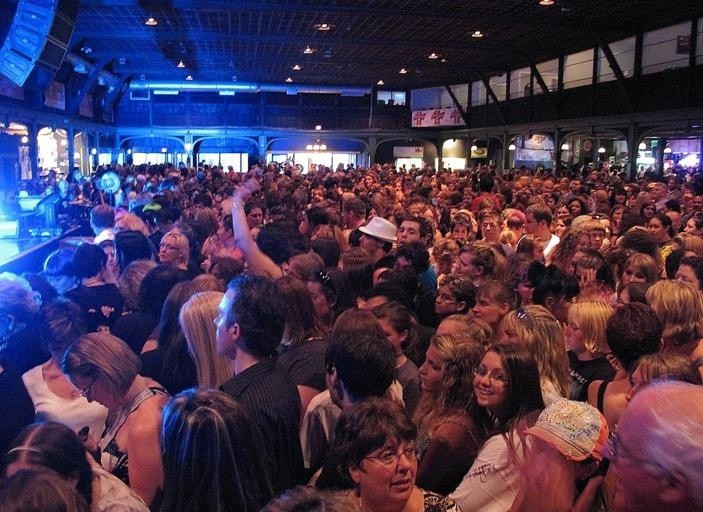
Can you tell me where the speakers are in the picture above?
[0,0,79,94]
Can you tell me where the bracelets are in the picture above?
[234,204,243,209]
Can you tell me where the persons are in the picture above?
[0,156,703,512]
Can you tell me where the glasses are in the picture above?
[360,444,422,465]
[316,270,334,287]
[603,421,669,478]
[159,242,181,250]
[76,375,102,400]
[514,304,535,327]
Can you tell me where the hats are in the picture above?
[358,216,398,244]
[523,397,611,463]
